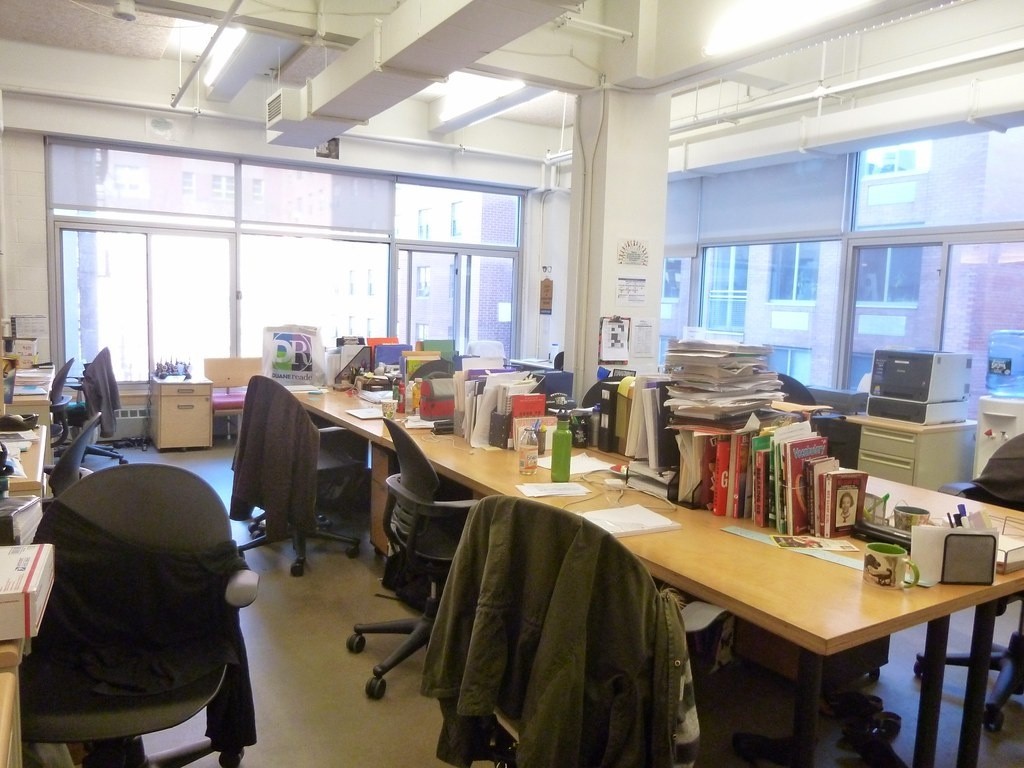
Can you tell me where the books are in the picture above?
[662,336,790,420]
[466,370,547,448]
[642,387,677,470]
[400,349,441,386]
[705,420,870,539]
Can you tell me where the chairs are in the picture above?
[345,416,479,701]
[935,432,1024,512]
[26,463,258,768]
[38,347,128,514]
[224,375,360,578]
[418,494,732,767]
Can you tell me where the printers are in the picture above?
[867,347,974,426]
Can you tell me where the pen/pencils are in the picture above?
[570,413,578,424]
[958,504,966,515]
[868,494,890,511]
[947,513,954,528]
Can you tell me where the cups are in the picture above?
[381,399,398,422]
[894,506,930,532]
[862,542,920,591]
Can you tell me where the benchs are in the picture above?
[205,356,261,442]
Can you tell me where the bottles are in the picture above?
[549,344,558,363]
[985,329,1024,400]
[551,406,572,482]
[393,374,422,416]
[519,427,539,475]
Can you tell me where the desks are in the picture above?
[151,373,213,452]
[285,373,1024,768]
[510,359,560,373]
[0,364,59,768]
[765,400,976,501]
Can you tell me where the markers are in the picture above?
[953,514,962,525]
